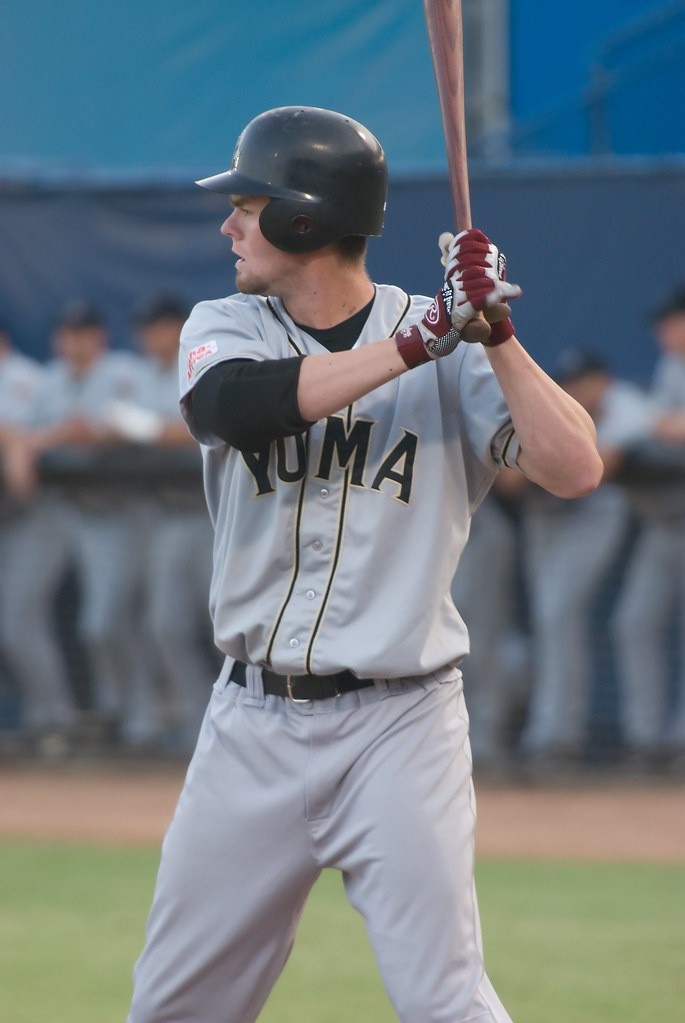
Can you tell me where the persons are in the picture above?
[125,107,604,1022]
[0,296,684,777]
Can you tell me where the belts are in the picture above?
[229,659,374,698]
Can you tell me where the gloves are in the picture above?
[396,228,523,371]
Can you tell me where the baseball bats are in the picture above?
[423,1,496,343]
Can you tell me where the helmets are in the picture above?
[645,284,684,320]
[195,105,388,255]
[127,289,190,325]
[48,296,105,329]
[549,340,613,386]
